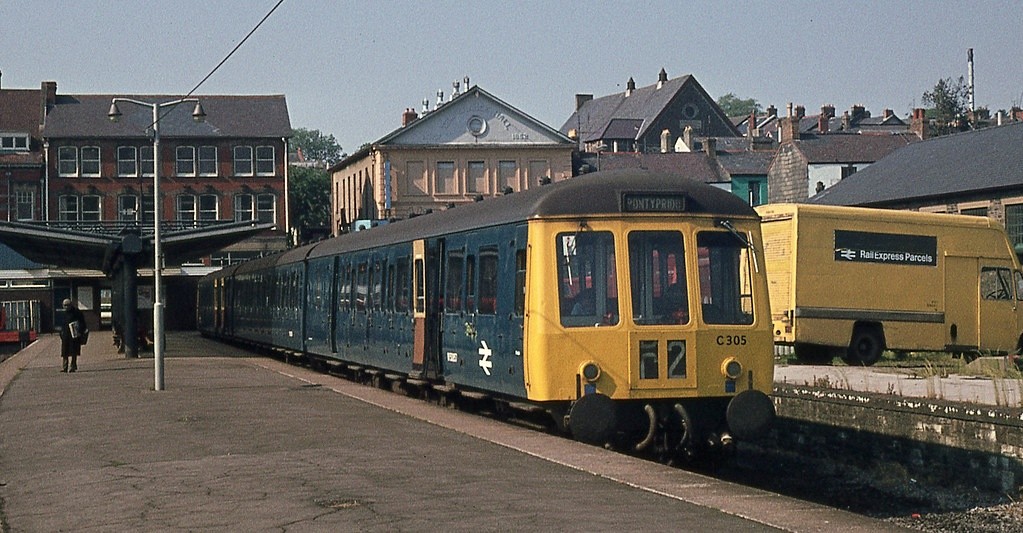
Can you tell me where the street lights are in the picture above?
[108,98,207,390]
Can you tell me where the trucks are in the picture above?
[740,203,1023,369]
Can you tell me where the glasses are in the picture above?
[63,304,71,307]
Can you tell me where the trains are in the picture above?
[195,168,775,455]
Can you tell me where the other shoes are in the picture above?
[69,367,75,373]
[60,367,67,372]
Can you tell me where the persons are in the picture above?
[59,299,88,373]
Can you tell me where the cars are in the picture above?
[101,304,112,318]
